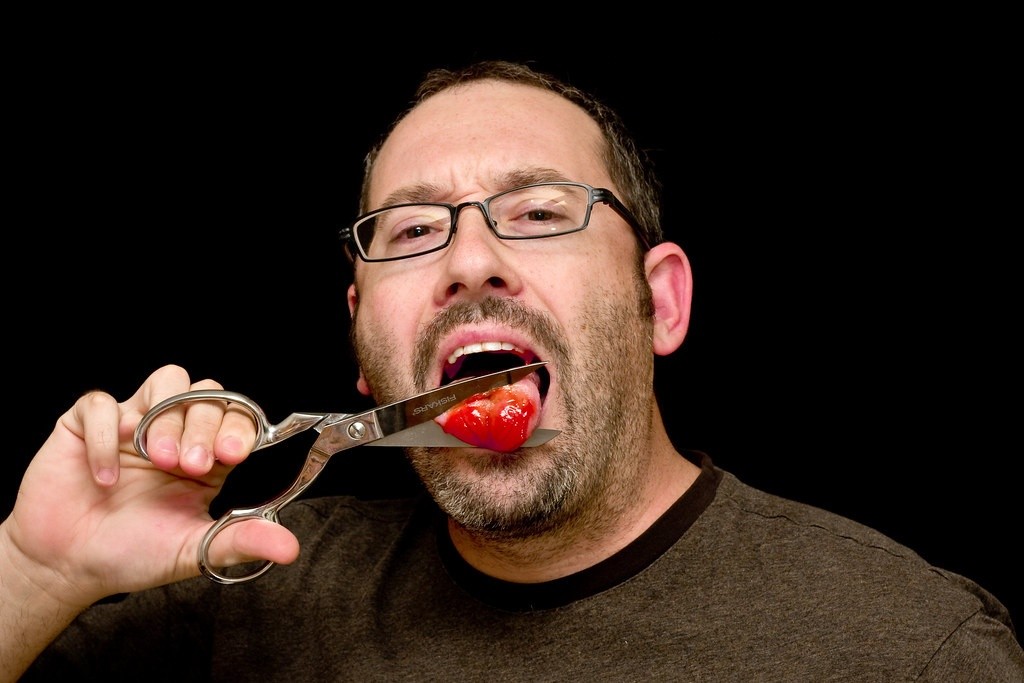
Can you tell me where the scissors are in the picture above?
[131,359,561,585]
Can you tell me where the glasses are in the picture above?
[340,182,651,262]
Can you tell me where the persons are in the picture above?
[0,56,1024,683]
